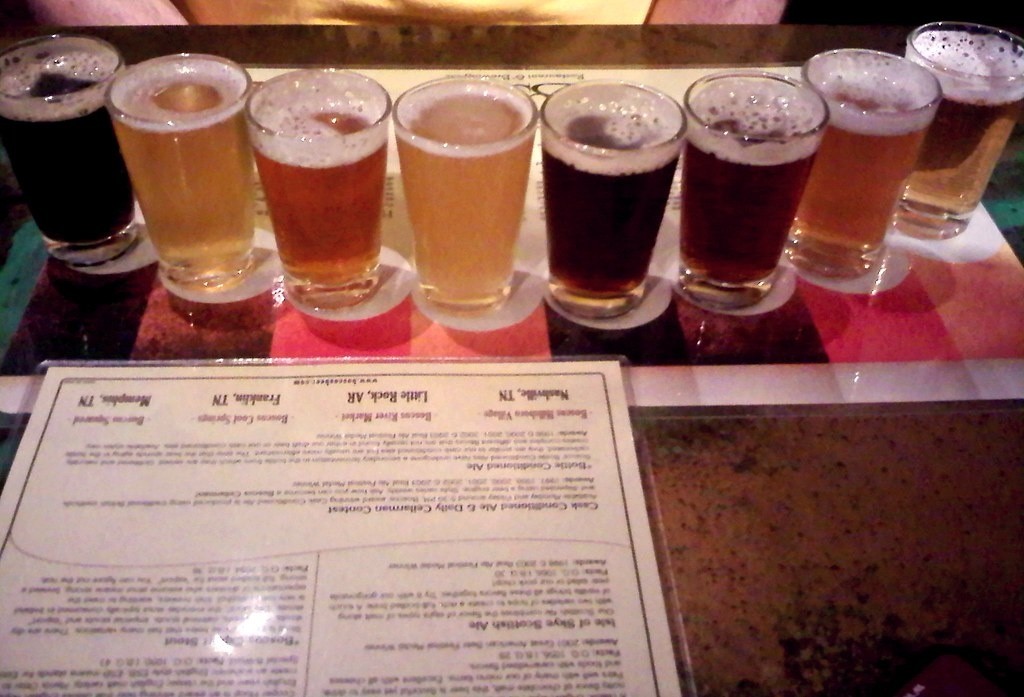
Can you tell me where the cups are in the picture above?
[243,65,392,309]
[887,19,1023,241]
[0,35,143,266]
[104,52,263,292]
[537,81,688,321]
[391,76,539,305]
[681,70,830,309]
[781,48,939,280]
